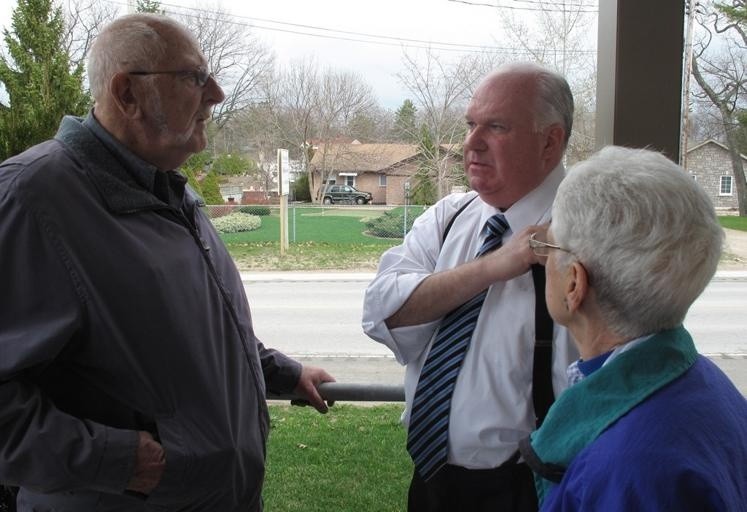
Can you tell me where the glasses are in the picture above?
[530,229,592,286]
[131,67,209,87]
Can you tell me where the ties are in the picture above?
[406,216,510,482]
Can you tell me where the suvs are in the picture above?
[318,185,372,204]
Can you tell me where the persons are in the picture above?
[361,60,581,510]
[517,142,746,511]
[0,11,338,511]
